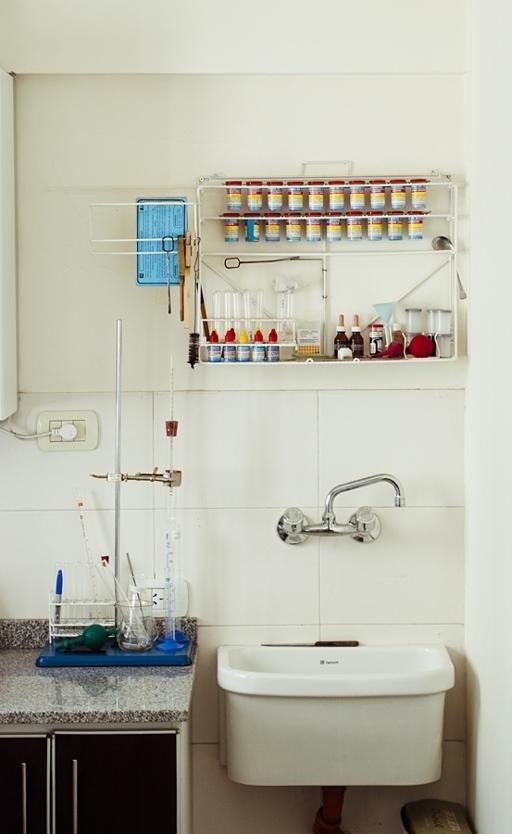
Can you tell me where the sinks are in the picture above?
[215,643,456,788]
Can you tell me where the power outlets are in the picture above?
[36,409,98,450]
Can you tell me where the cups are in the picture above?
[113,600,159,651]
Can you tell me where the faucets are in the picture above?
[324,474,405,515]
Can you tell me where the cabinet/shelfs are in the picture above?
[0,724,189,834]
[197,181,459,369]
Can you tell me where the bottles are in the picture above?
[208,328,279,361]
[334,313,363,358]
[368,323,384,354]
